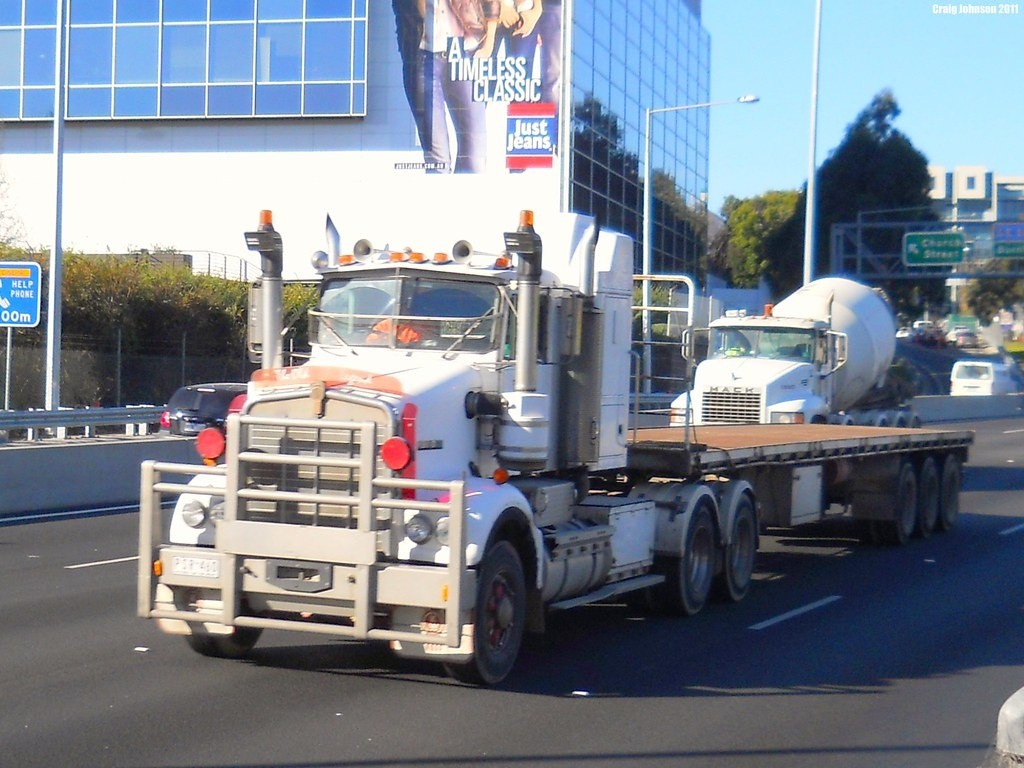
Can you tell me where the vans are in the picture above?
[156,383,250,438]
[948,361,1019,396]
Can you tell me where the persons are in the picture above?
[724,338,752,356]
[365,303,423,345]
[392,0,561,174]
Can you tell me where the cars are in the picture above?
[913,321,934,330]
[949,328,976,348]
[895,326,914,337]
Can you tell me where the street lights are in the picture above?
[642,96,761,402]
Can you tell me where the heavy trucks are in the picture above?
[671,278,921,429]
[138,209,979,688]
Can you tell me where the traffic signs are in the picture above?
[903,230,966,266]
